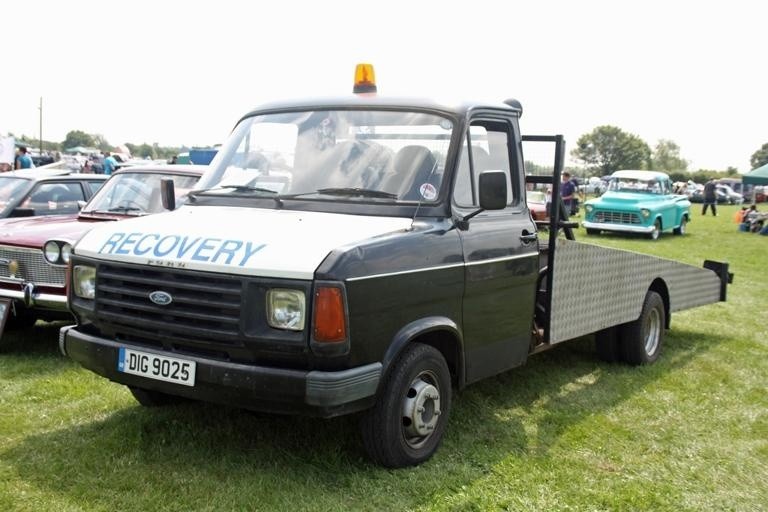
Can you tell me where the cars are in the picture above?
[525,169,745,239]
[1,165,209,338]
[1,163,110,221]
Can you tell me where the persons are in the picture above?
[562,172,575,221]
[167,156,178,164]
[16,147,31,169]
[85,151,118,175]
[702,177,718,216]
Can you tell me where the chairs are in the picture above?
[376,144,438,201]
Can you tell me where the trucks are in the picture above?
[56,61,737,469]
